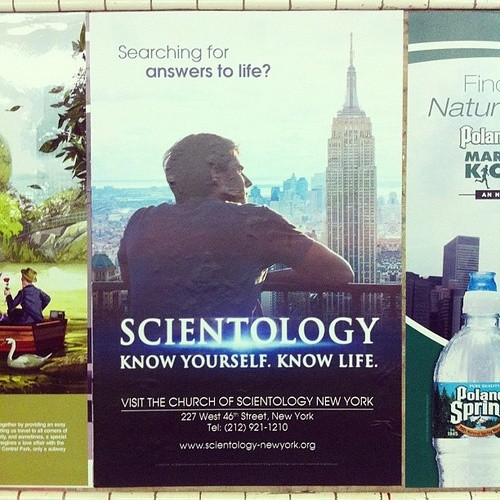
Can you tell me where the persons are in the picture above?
[114,129,358,320]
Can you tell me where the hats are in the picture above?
[21,268,38,282]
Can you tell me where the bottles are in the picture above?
[431,271,500,488]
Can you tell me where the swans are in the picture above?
[0,337,53,369]
